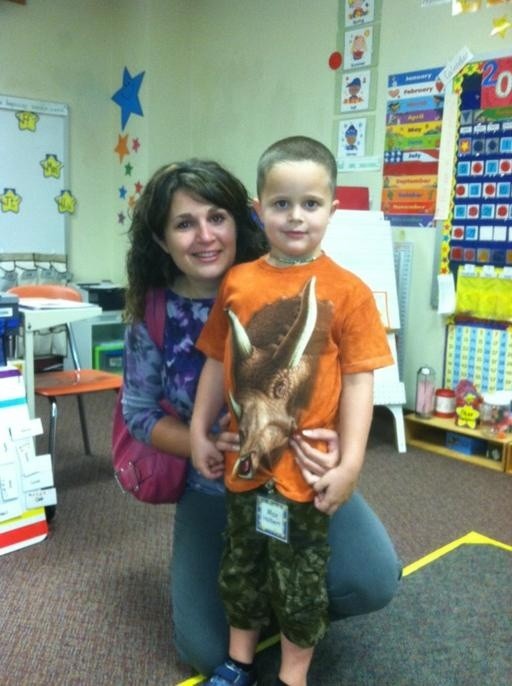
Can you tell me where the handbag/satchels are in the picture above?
[112,387,188,504]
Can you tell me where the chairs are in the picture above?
[5,284,124,475]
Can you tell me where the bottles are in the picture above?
[479,391,512,440]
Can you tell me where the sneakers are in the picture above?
[202,658,259,686]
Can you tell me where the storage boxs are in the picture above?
[79,281,126,310]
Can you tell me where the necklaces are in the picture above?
[269,250,320,265]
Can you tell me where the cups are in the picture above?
[414,365,435,420]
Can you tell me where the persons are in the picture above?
[186,136,393,686]
[122,159,402,673]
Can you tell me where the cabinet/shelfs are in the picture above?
[63,307,128,370]
[403,412,512,471]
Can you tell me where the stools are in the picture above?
[94,339,126,375]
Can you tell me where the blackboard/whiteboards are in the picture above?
[0,93,71,262]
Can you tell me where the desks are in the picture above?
[17,295,102,450]
